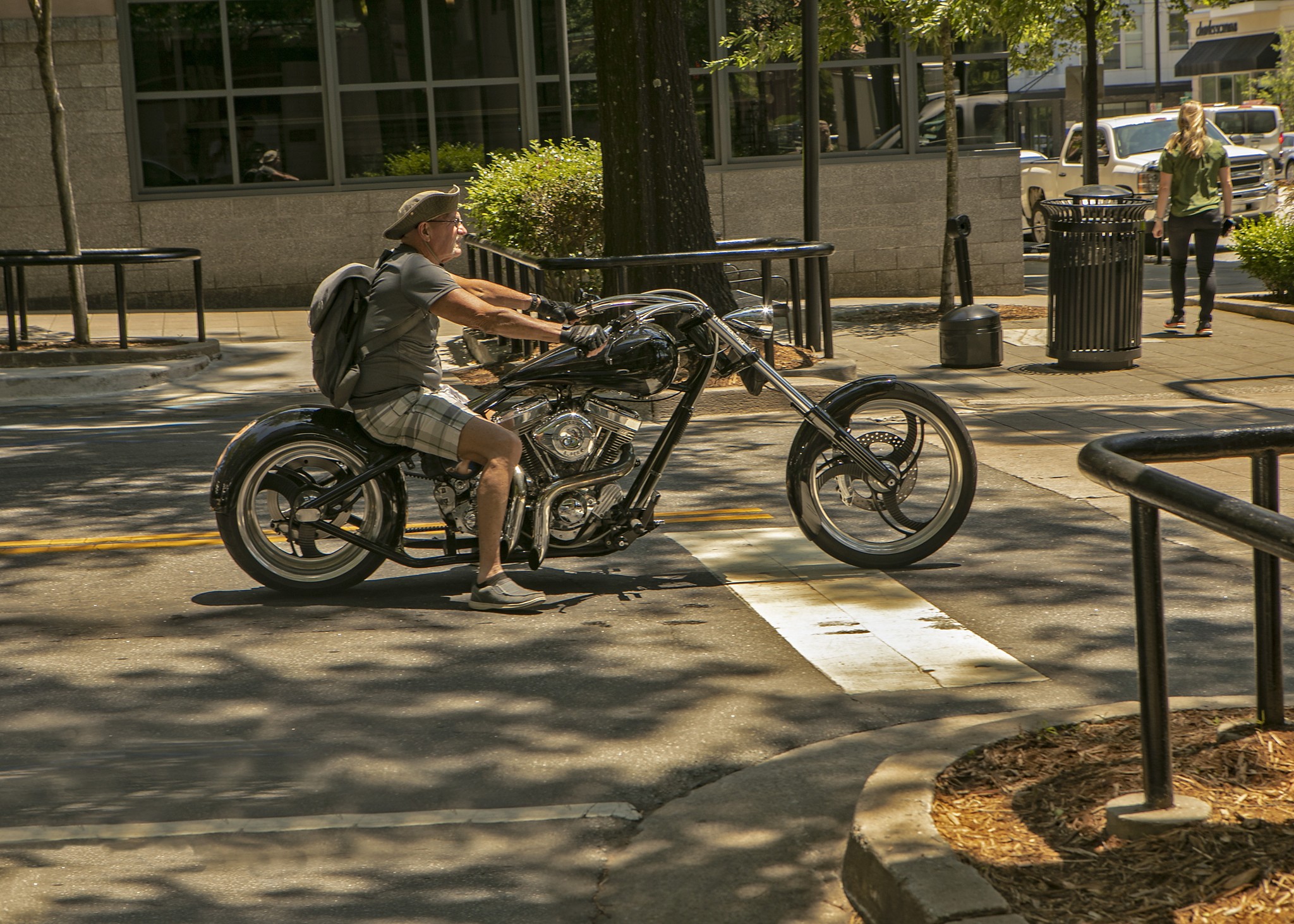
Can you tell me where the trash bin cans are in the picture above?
[1044,197,1153,369]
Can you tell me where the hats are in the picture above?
[259,149,280,164]
[382,184,460,240]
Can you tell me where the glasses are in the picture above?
[416,217,463,228]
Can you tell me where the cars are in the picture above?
[1283,131,1294,185]
[1016,149,1048,234]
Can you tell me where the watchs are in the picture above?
[1153,215,1163,223]
[526,292,539,314]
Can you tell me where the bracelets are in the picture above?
[1223,215,1230,219]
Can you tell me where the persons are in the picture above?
[1152,101,1235,336]
[308,185,611,611]
[819,119,836,152]
[245,148,299,181]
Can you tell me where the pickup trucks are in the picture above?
[1020,111,1282,250]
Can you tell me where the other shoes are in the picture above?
[467,577,546,610]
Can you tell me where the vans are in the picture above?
[868,88,1008,150]
[1161,102,1286,175]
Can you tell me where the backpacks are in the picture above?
[241,168,261,183]
[308,249,429,409]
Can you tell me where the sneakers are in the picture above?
[1163,315,1186,328]
[1193,320,1213,336]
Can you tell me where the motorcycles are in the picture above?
[208,289,980,592]
